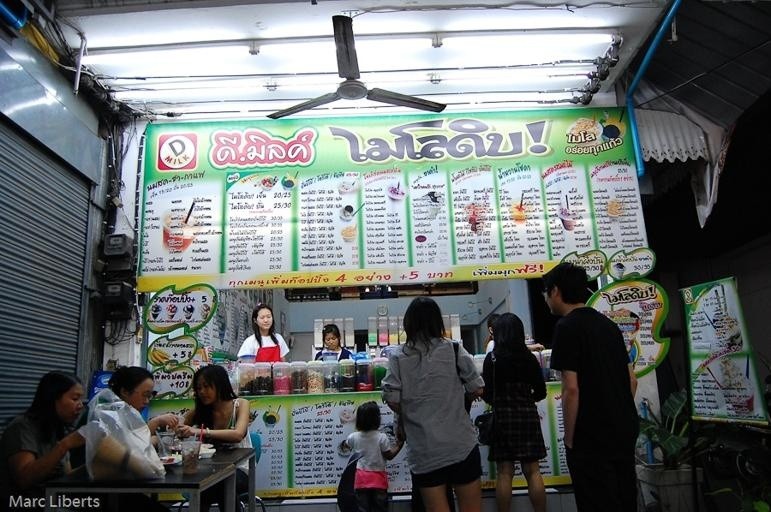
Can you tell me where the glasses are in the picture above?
[540,287,548,296]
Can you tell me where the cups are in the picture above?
[339,410,356,424]
[606,200,625,218]
[340,208,355,222]
[260,176,273,192]
[557,210,580,232]
[340,226,358,243]
[337,181,360,195]
[728,394,758,418]
[181,440,202,474]
[161,213,201,252]
[384,185,408,213]
[426,199,444,222]
[337,447,352,457]
[613,265,626,278]
[282,176,297,190]
[263,411,280,428]
[719,330,744,354]
[158,430,176,457]
[511,202,527,224]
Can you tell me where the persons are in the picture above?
[237,302,291,362]
[485,313,500,356]
[539,260,641,512]
[174,363,258,511]
[0,370,87,490]
[343,400,405,512]
[315,324,355,360]
[381,295,486,512]
[87,365,179,511]
[480,312,548,512]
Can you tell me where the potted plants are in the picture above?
[635,388,704,512]
[704,486,771,512]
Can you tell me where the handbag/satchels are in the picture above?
[474,412,498,446]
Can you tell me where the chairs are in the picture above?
[240,432,266,512]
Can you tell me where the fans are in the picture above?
[267,14,446,119]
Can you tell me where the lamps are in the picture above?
[466,297,492,307]
[461,308,482,320]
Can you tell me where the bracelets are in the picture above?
[205,426,210,440]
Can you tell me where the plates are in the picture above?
[161,442,215,466]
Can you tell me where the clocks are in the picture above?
[376,305,389,316]
